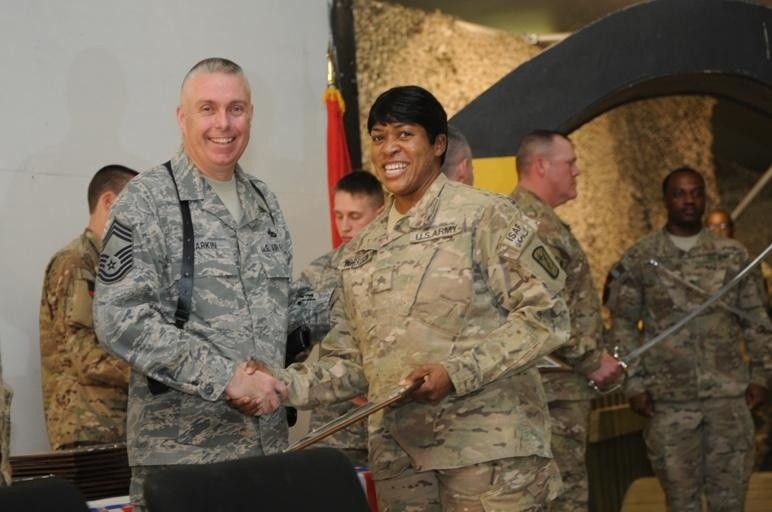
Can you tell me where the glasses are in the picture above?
[708,222,732,231]
